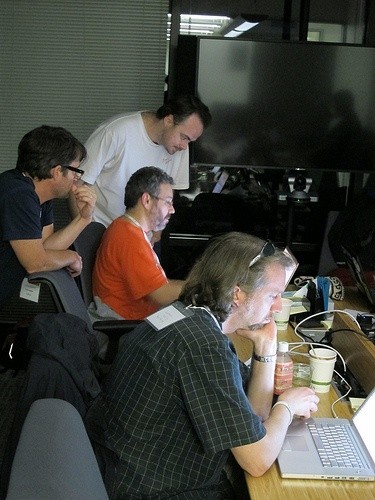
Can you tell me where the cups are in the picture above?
[273,298,293,331]
[307,348,336,393]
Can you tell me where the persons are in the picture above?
[0,123,99,309]
[93,232,322,500]
[67,93,212,308]
[92,166,186,319]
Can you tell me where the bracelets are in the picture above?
[272,401,294,426]
[252,351,278,363]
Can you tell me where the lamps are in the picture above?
[221,13,265,38]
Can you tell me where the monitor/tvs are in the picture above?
[173,34,375,175]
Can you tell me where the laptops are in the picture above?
[211,170,230,194]
[275,387,375,482]
[282,245,299,290]
[339,245,375,307]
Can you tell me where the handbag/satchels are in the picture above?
[0,326,33,371]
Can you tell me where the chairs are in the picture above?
[0,185,375,500]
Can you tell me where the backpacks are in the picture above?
[185,192,274,235]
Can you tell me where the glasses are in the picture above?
[248,238,276,268]
[52,164,84,180]
[151,194,173,207]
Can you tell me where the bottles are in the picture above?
[275,342,294,396]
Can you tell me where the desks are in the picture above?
[227,299,375,500]
